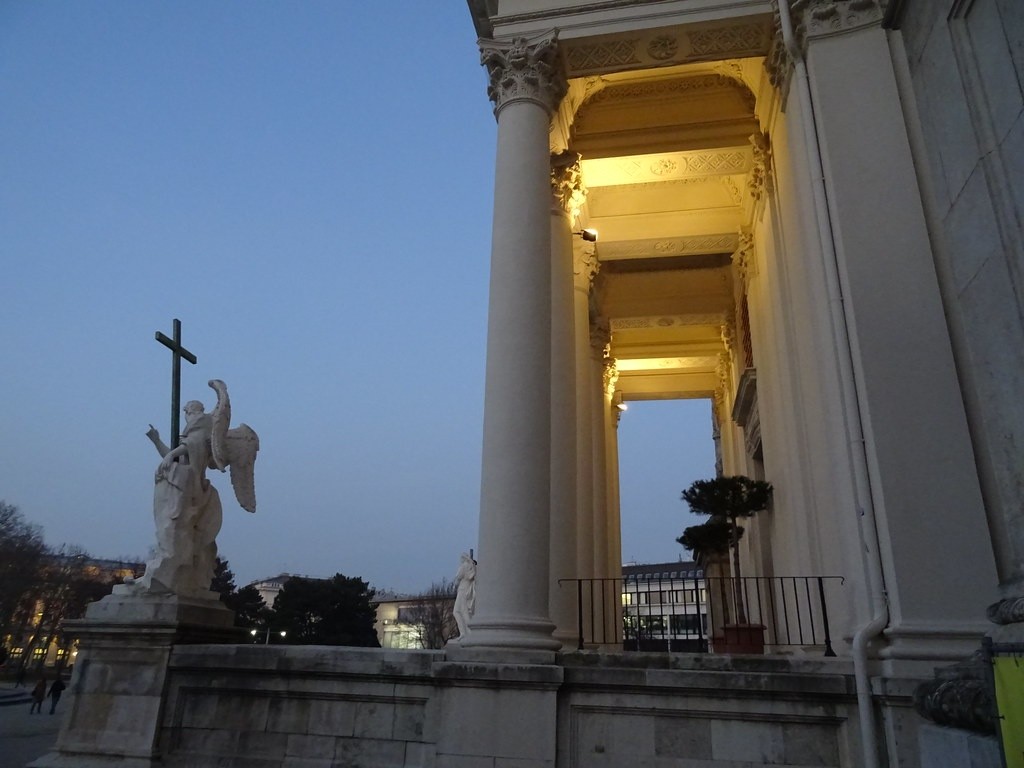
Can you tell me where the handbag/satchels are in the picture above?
[30,686,38,697]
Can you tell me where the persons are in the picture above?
[447,552,478,645]
[30,676,48,715]
[46,677,66,714]
[112,378,259,598]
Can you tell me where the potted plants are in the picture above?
[675,523,745,655]
[679,475,774,655]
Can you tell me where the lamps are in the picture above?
[615,390,628,411]
[573,228,598,242]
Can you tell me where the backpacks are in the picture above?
[57,681,65,690]
